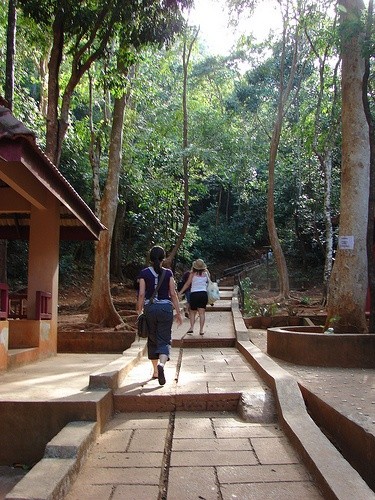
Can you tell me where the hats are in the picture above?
[193,259,207,270]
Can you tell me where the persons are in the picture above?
[137,246,183,385]
[178,259,210,335]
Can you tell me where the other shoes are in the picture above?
[153,373,158,379]
[200,333,204,335]
[187,331,193,333]
[157,364,165,385]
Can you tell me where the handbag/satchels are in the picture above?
[136,313,149,338]
[206,272,220,305]
